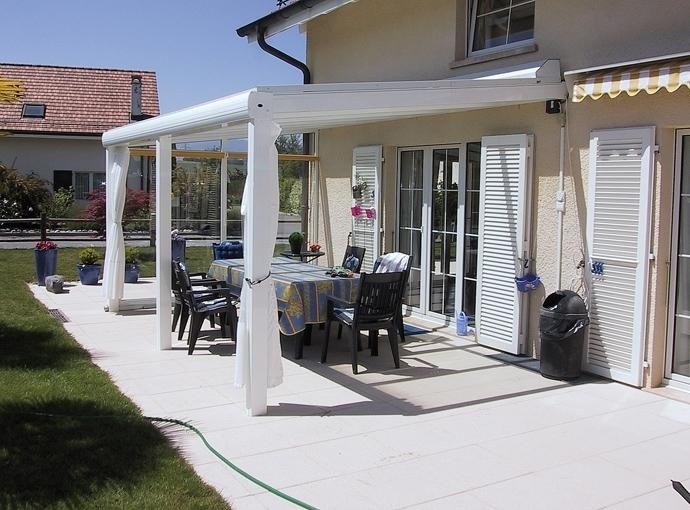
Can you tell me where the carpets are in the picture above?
[331,309,434,337]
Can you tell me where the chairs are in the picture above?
[170,257,237,355]
[320,245,413,376]
[212,240,243,260]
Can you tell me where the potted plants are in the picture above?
[125,246,140,284]
[76,248,101,284]
[288,232,303,253]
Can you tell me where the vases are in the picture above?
[34,249,58,286]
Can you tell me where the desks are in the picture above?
[208,257,361,359]
[280,249,325,263]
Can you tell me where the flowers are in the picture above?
[35,240,60,251]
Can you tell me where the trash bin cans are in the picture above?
[538,290,588,381]
